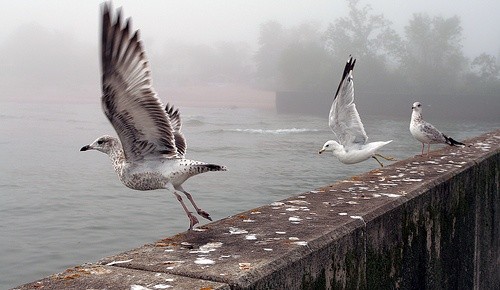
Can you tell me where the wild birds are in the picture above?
[409,102,466,159]
[79,0,228,230]
[318,57,398,168]
